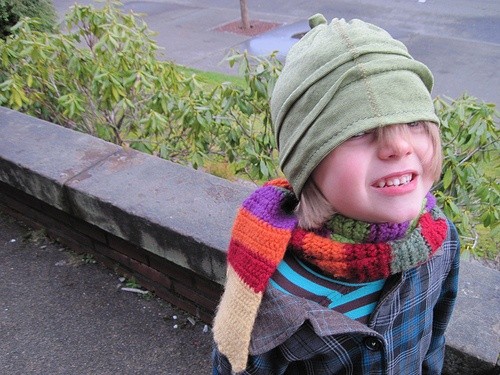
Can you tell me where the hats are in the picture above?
[268,14,443,198]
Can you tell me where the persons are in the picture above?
[210,11,462,374]
